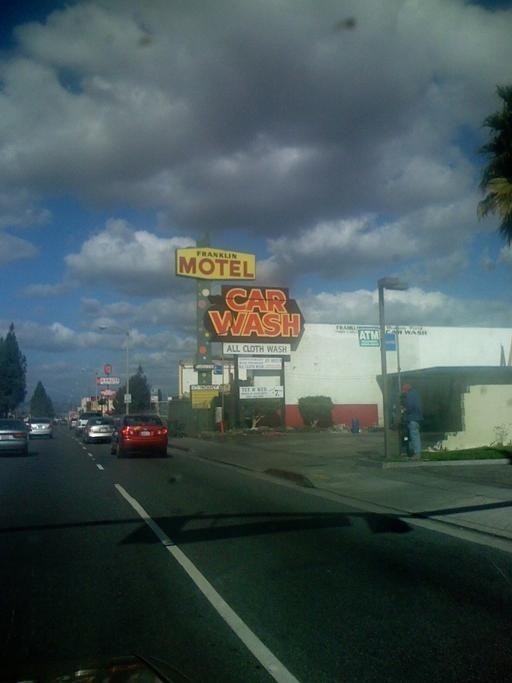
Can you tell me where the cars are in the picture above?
[28,413,52,438]
[52,406,117,443]
[112,410,170,462]
[0,416,30,457]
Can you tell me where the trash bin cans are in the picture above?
[218,421,224,432]
[352,419,358,432]
[168,422,174,431]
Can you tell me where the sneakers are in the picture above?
[408,454,420,460]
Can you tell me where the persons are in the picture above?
[401,382,425,460]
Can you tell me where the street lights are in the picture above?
[96,323,131,414]
